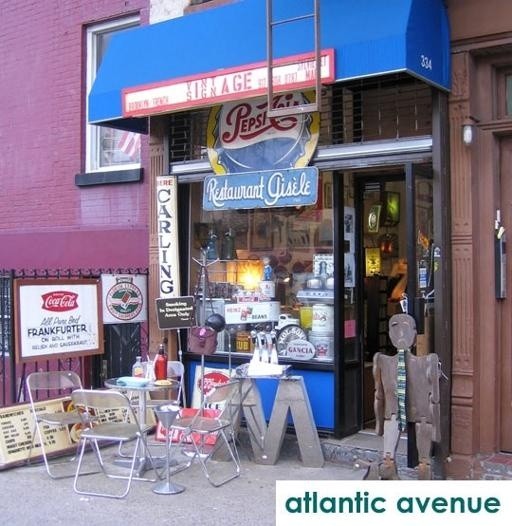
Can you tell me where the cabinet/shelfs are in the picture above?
[250,326,280,368]
[206,235,234,260]
[132,344,168,380]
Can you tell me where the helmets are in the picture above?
[152,380,173,385]
[118,376,151,386]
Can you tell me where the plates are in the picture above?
[366,275,390,362]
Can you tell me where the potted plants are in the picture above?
[247,209,274,251]
[12,279,105,364]
[325,183,332,208]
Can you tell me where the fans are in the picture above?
[155,296,198,330]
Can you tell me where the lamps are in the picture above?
[26,361,243,499]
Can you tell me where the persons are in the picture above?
[367,310,451,480]
[262,258,273,281]
